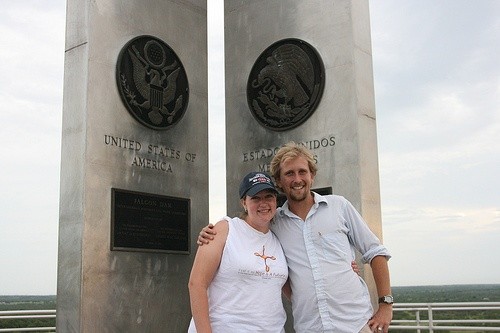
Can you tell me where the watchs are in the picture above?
[378,294,393,304]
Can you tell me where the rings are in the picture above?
[378,327,383,331]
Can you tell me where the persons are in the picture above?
[188,171,361,333]
[197,141,393,333]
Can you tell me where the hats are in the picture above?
[239,172,279,199]
